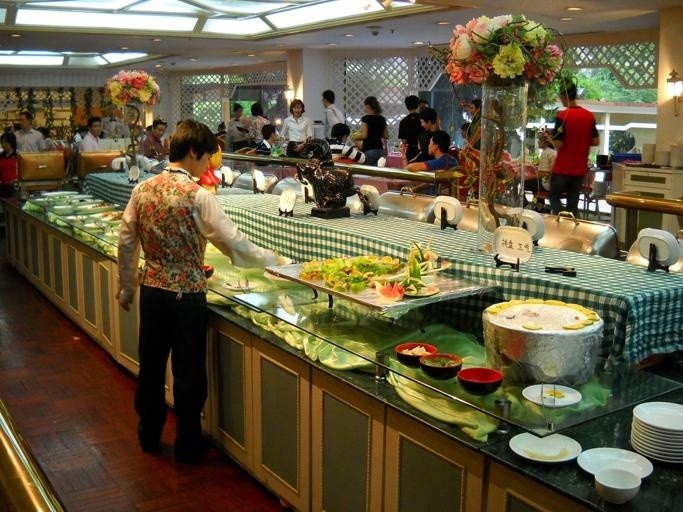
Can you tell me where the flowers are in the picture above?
[101,69,164,176]
[443,13,572,232]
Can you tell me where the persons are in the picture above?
[113,119,290,468]
[497,144,532,208]
[214,89,493,196]
[537,77,600,219]
[529,133,558,211]
[0,109,169,199]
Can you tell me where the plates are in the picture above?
[631,400,683,463]
[112,157,127,171]
[577,447,654,478]
[358,184,379,211]
[492,226,532,265]
[433,196,463,225]
[509,209,544,241]
[252,170,265,191]
[642,143,683,168]
[637,227,680,266]
[509,429,582,464]
[39,191,80,196]
[221,166,233,185]
[129,166,140,181]
[279,189,296,212]
[522,384,582,409]
[77,202,121,212]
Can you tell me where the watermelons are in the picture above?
[375,281,405,302]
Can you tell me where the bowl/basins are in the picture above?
[419,353,463,380]
[457,367,504,395]
[594,469,641,504]
[395,342,437,368]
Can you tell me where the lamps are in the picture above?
[666,69,682,121]
[283,85,292,99]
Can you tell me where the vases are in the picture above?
[477,82,531,253]
[122,99,148,180]
